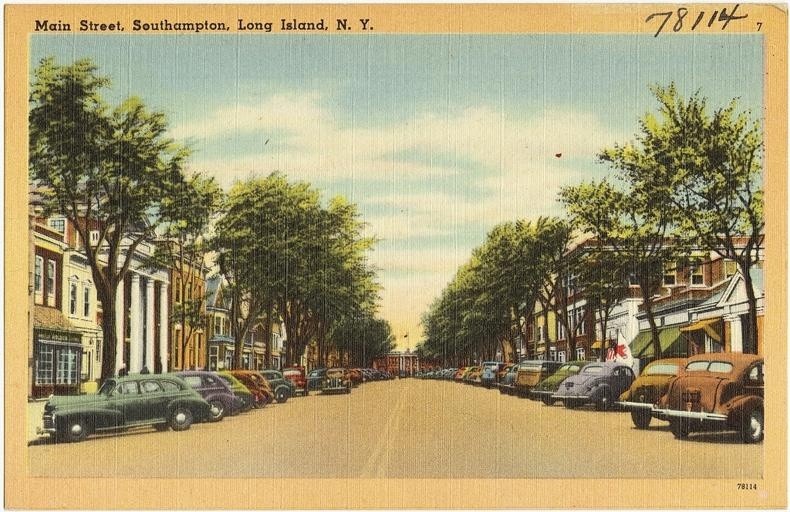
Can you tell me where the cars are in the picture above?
[307,368,395,394]
[614,352,763,444]
[40,368,308,441]
[416,362,636,410]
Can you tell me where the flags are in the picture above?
[616,329,635,369]
[605,339,617,360]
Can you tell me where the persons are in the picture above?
[140,364,149,374]
[119,363,129,376]
